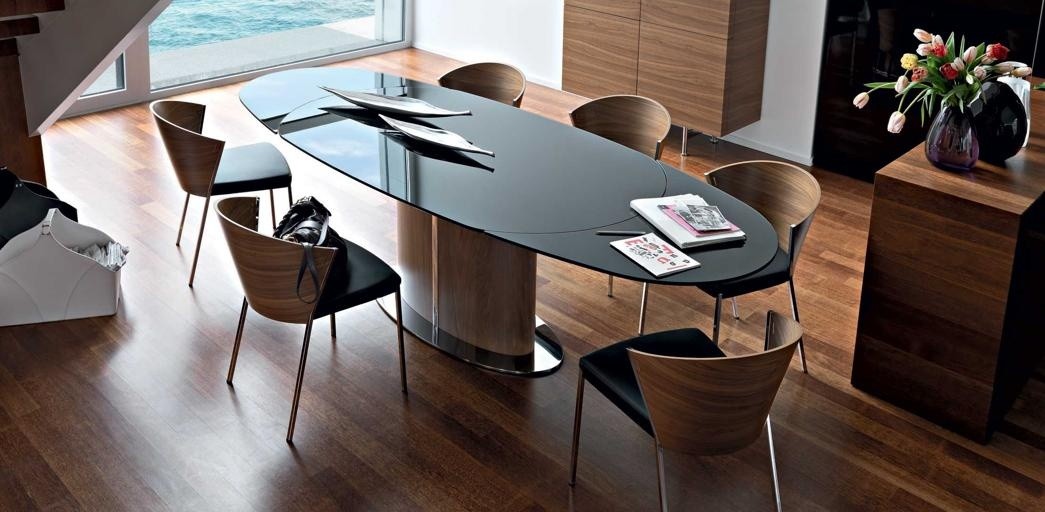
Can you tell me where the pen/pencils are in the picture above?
[596,231,645,234]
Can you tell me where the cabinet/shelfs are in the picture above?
[562,0,771,137]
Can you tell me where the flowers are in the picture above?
[853,26,1032,135]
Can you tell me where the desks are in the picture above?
[237,66,778,380]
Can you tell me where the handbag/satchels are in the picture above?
[272,195,348,304]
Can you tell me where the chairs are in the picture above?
[565,309,804,511]
[567,93,671,300]
[150,99,294,290]
[215,193,409,444]
[436,61,528,109]
[637,160,821,374]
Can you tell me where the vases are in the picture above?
[923,102,981,173]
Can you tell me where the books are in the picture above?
[608,230,704,277]
[66,239,130,273]
[628,191,748,250]
